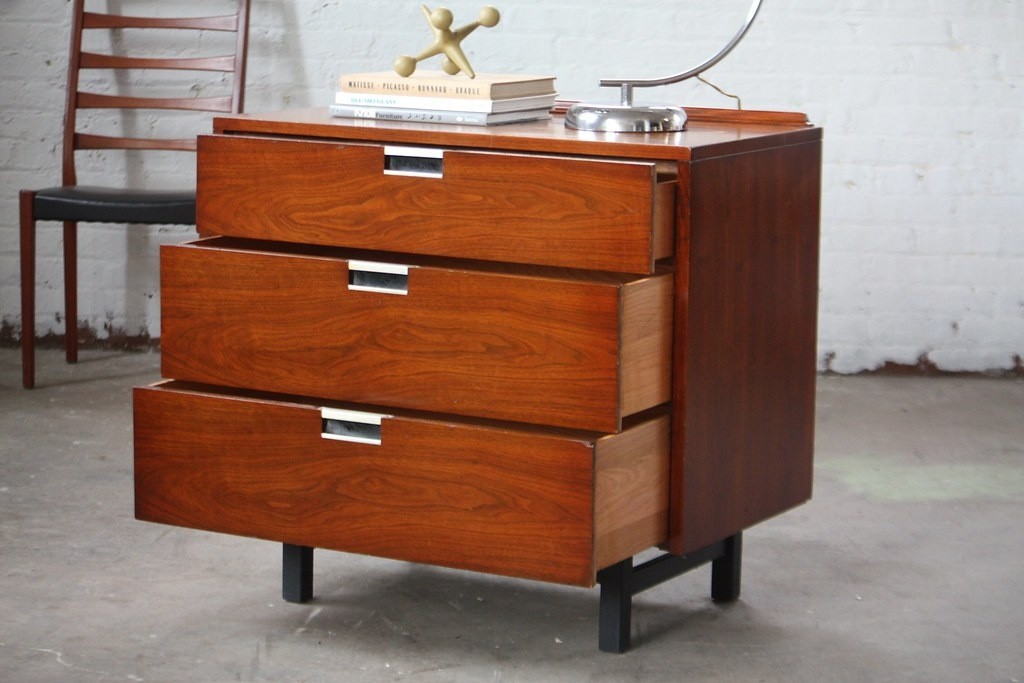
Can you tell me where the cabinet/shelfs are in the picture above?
[131,96,823,655]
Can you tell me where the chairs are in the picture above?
[20,0,252,391]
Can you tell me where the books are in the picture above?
[329,69,560,127]
